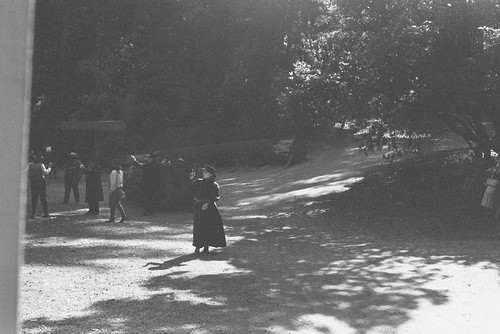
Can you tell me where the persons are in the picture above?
[190,165,226,254]
[129,154,166,216]
[27,153,53,217]
[80,160,103,215]
[105,160,127,222]
[478,151,500,236]
[63,152,82,204]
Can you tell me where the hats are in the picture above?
[202,165,216,179]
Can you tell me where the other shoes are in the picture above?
[195,249,199,255]
[83,211,99,214]
[202,250,208,255]
[105,218,115,223]
[120,216,127,222]
